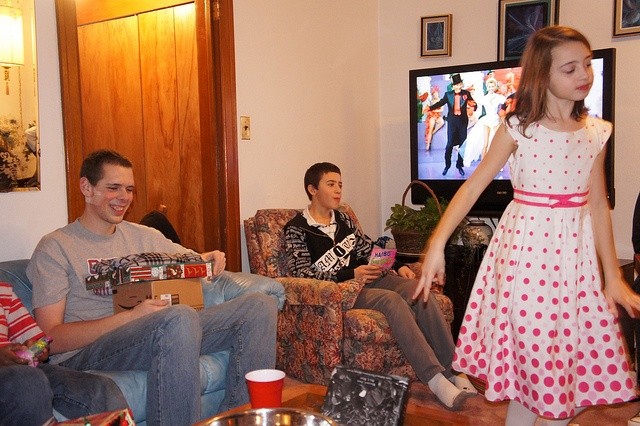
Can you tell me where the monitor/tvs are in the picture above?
[409,48,615,212]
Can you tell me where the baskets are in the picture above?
[391,180,443,255]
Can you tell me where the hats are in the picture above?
[449,73,464,85]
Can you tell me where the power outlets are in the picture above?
[239,115,251,140]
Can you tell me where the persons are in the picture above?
[458,79,505,167]
[424,74,478,176]
[498,91,516,119]
[282,162,477,411]
[25,149,278,426]
[500,69,516,93]
[483,70,499,96]
[412,25,636,426]
[1,281,136,426]
[425,87,444,151]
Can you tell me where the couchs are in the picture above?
[0,260,287,426]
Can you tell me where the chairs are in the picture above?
[243,203,455,385]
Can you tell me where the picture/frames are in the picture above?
[612,0,640,38]
[497,0,560,62]
[419,14,452,57]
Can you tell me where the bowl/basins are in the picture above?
[203,408,333,426]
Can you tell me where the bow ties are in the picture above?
[455,92,461,95]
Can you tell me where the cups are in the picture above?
[244,368,286,410]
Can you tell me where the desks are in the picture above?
[204,376,470,426]
[387,243,634,363]
